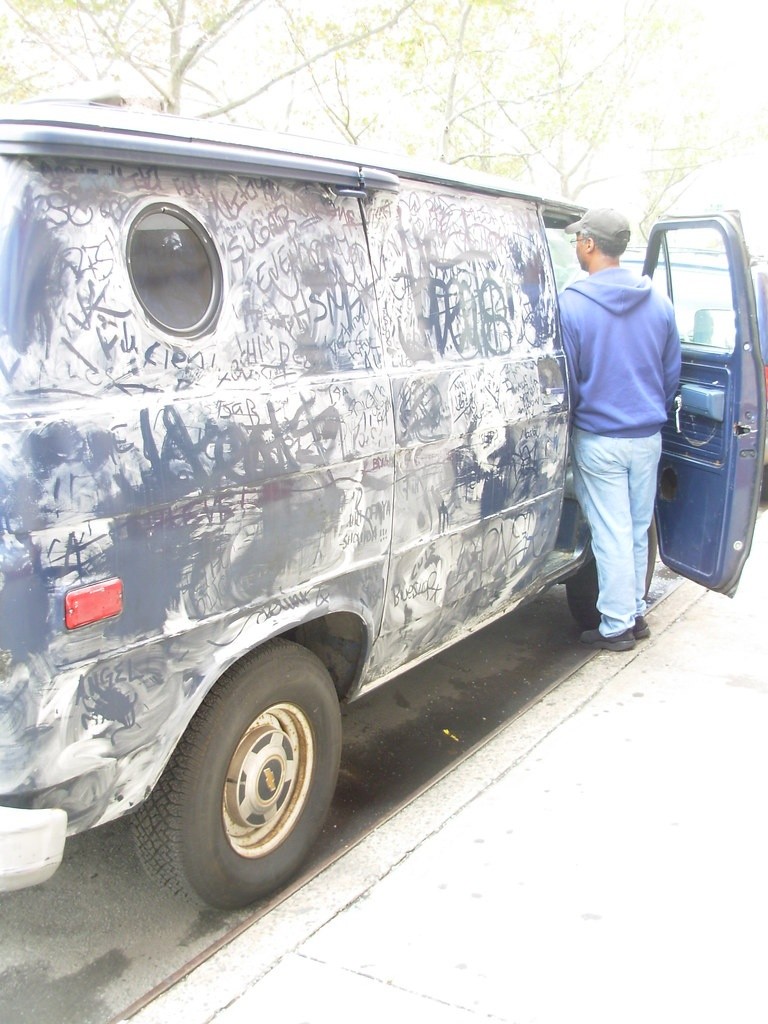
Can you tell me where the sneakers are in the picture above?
[632,616,650,638]
[578,629,635,651]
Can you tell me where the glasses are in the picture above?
[570,238,586,247]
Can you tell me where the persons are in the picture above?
[558,206,681,650]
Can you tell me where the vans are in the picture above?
[2,107,768,904]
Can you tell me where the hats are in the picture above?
[565,207,631,243]
